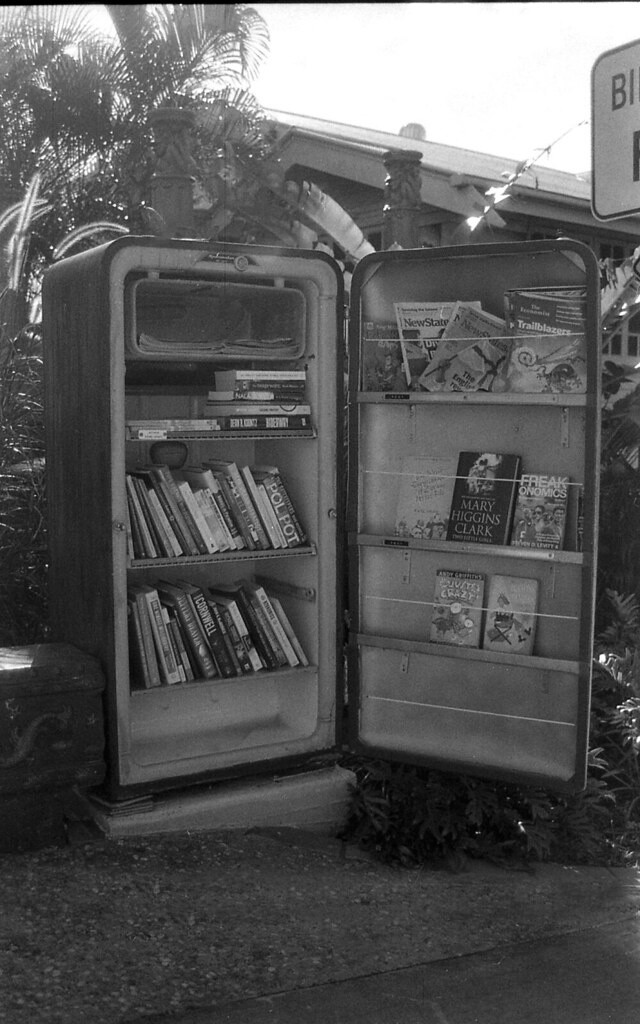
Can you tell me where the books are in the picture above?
[362,286,589,653]
[126,309,313,692]
